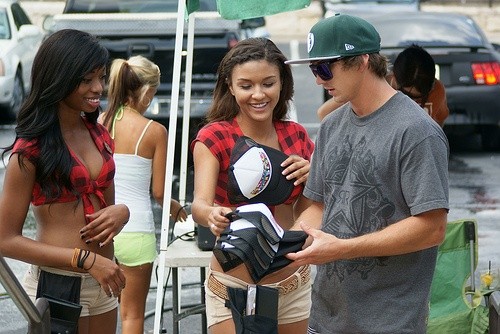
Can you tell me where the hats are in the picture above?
[213,241,286,284]
[284,14,381,64]
[220,218,305,267]
[224,203,307,245]
[227,136,296,206]
[217,234,287,277]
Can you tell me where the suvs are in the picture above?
[42,0,267,121]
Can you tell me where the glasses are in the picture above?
[399,89,422,99]
[309,57,346,81]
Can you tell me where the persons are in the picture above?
[97,55,188,334]
[283,13,450,334]
[0,28,131,334]
[191,38,316,334]
[316,44,449,129]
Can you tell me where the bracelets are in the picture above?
[73,247,97,271]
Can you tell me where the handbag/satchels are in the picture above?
[36,294,83,334]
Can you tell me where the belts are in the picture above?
[207,264,312,301]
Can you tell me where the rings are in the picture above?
[210,224,215,229]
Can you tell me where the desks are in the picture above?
[157,235,214,334]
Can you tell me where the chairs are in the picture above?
[425,220,495,333]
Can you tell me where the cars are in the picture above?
[0,0,49,122]
[364,13,499,154]
